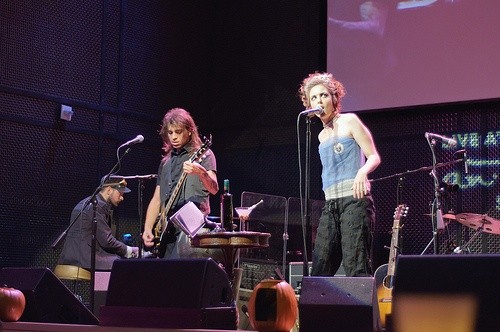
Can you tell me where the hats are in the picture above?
[100,174,131,193]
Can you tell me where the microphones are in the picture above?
[137,174,158,180]
[464,152,469,174]
[440,182,459,191]
[123,134,145,146]
[301,105,323,115]
[427,132,457,146]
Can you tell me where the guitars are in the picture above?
[374,203,409,328]
[150,134,213,260]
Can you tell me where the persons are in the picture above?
[57,176,139,270]
[142,108,219,257]
[299,73,381,277]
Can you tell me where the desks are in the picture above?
[190,231,271,279]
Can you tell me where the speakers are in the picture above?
[0,268,101,325]
[297,275,383,331]
[105,259,239,331]
[391,253,500,332]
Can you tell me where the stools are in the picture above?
[55,265,91,295]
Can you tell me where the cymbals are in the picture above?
[455,213,500,234]
[422,213,458,220]
